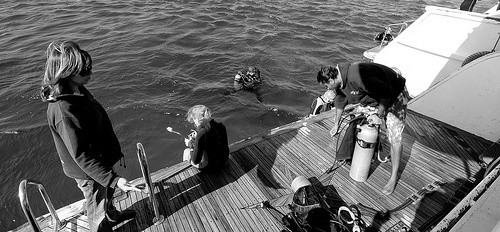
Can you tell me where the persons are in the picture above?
[40,40,142,232]
[378,25,395,45]
[182,103,229,173]
[233,66,261,87]
[316,62,410,196]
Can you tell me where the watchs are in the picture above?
[374,113,384,119]
